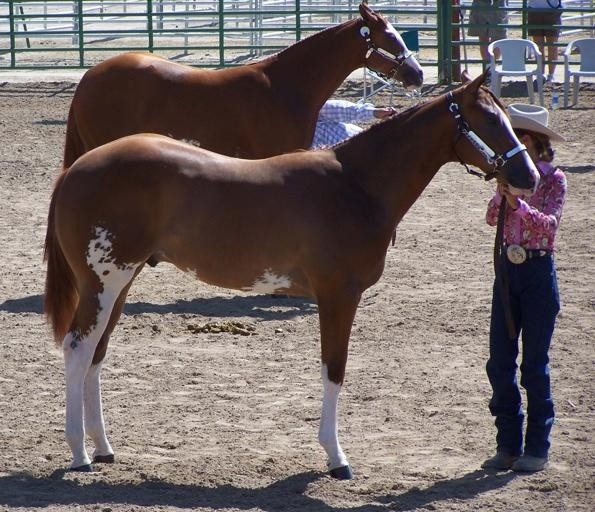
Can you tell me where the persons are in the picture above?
[467,0,513,79]
[525,0,565,84]
[311,98,401,152]
[482,104,568,473]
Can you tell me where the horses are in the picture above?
[40,64,543,481]
[62,0,424,170]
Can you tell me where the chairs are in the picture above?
[486,34,547,111]
[561,29,595,109]
[362,28,420,101]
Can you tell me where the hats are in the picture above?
[506,104,566,142]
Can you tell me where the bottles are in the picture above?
[551,90,560,110]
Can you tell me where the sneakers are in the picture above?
[481,450,550,473]
[533,73,556,84]
